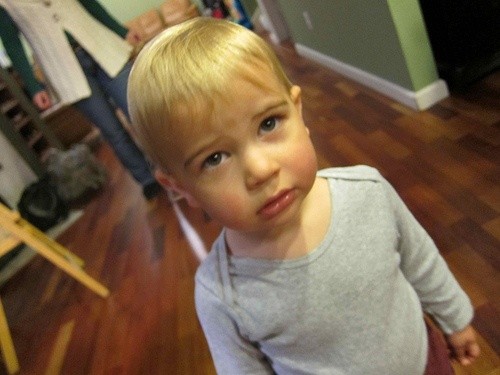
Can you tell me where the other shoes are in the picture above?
[143,182,162,202]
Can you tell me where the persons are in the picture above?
[125,16,480,375]
[0,0,163,199]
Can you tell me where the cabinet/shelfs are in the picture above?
[0,65,105,180]
